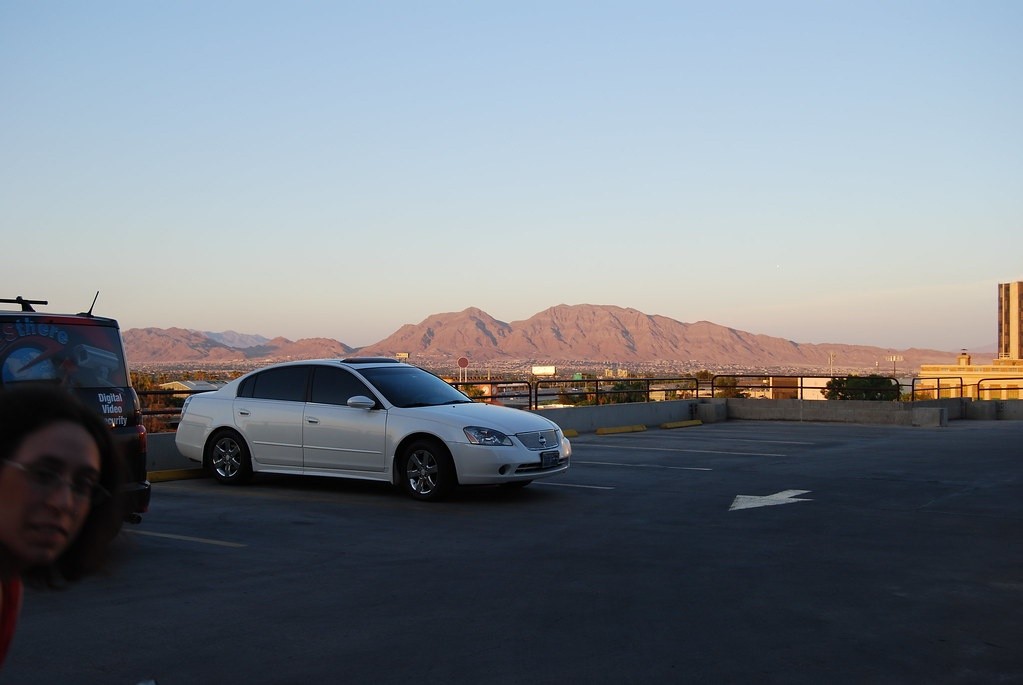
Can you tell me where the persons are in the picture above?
[0,387,119,668]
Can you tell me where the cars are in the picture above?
[175,356,574,503]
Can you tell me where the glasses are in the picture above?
[0,456,99,498]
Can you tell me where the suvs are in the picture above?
[0,293,153,562]
